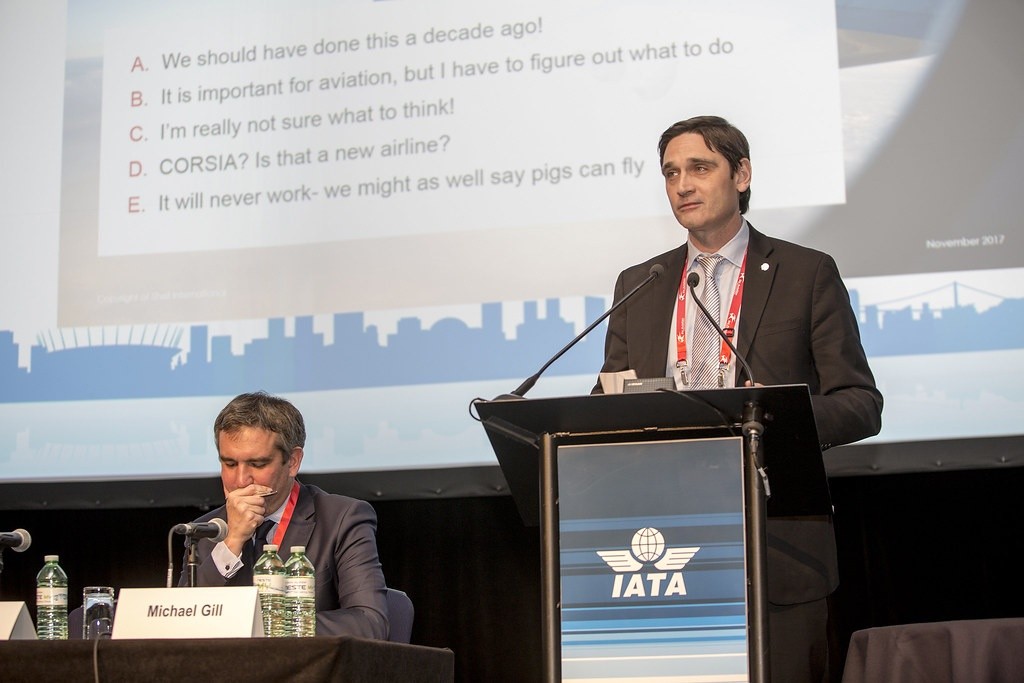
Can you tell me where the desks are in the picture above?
[841,617,1024,683]
[0,633,454,683]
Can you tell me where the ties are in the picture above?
[252,521,274,565]
[689,254,726,391]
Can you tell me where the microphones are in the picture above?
[175,518,229,543]
[492,265,665,401]
[0,529,32,552]
[687,272,755,387]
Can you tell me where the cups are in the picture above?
[83,587,114,640]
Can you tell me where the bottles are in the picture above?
[253,545,287,637]
[285,546,316,638]
[36,556,68,640]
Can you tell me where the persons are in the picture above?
[176,389,389,639]
[594,116,884,683]
